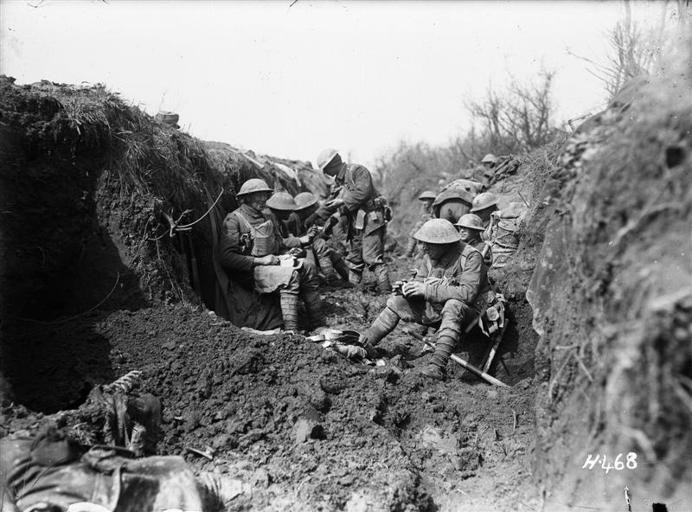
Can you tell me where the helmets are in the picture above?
[468,192,501,213]
[293,191,319,211]
[417,190,436,200]
[411,218,461,246]
[316,146,340,170]
[236,178,273,198]
[452,213,487,232]
[265,191,298,212]
[480,153,498,164]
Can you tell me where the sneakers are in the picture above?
[419,366,446,379]
